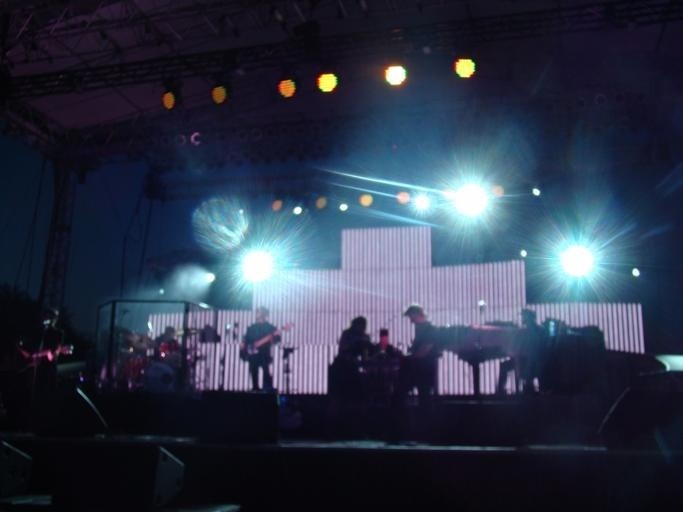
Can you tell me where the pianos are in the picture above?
[442,324,605,394]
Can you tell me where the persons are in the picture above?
[238,305,282,392]
[153,326,176,361]
[492,307,547,395]
[390,302,443,410]
[24,307,65,390]
[328,315,376,396]
[368,326,404,359]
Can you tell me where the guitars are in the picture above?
[239,322,292,361]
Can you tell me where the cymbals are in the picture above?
[175,329,197,336]
[127,332,154,351]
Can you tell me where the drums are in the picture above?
[123,356,144,387]
[145,357,182,394]
[159,341,182,363]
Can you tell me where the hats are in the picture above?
[405,306,422,315]
